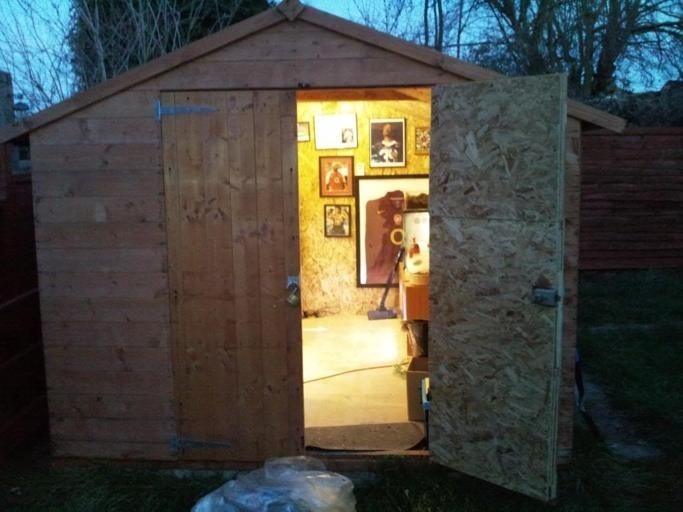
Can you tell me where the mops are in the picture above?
[366,245,406,323]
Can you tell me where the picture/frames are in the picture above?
[295,111,431,287]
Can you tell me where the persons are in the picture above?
[325,163,347,190]
[327,205,348,235]
[372,123,402,163]
